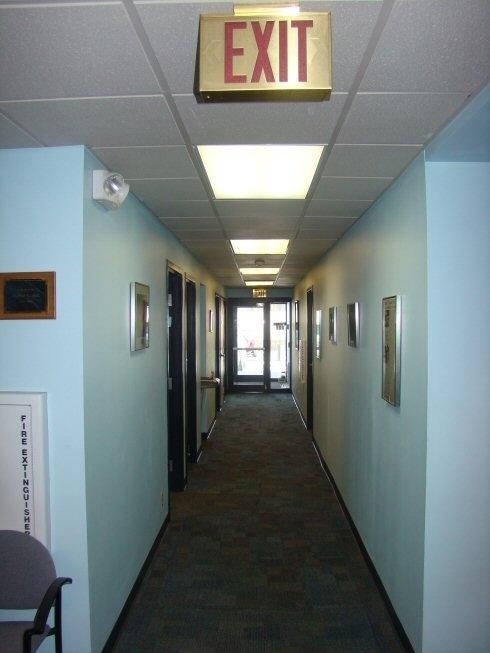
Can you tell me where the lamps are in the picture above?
[91,167,131,211]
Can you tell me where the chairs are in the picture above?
[0,529,72,652]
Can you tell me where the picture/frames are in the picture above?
[131,281,151,351]
[312,300,360,359]
[380,294,401,408]
